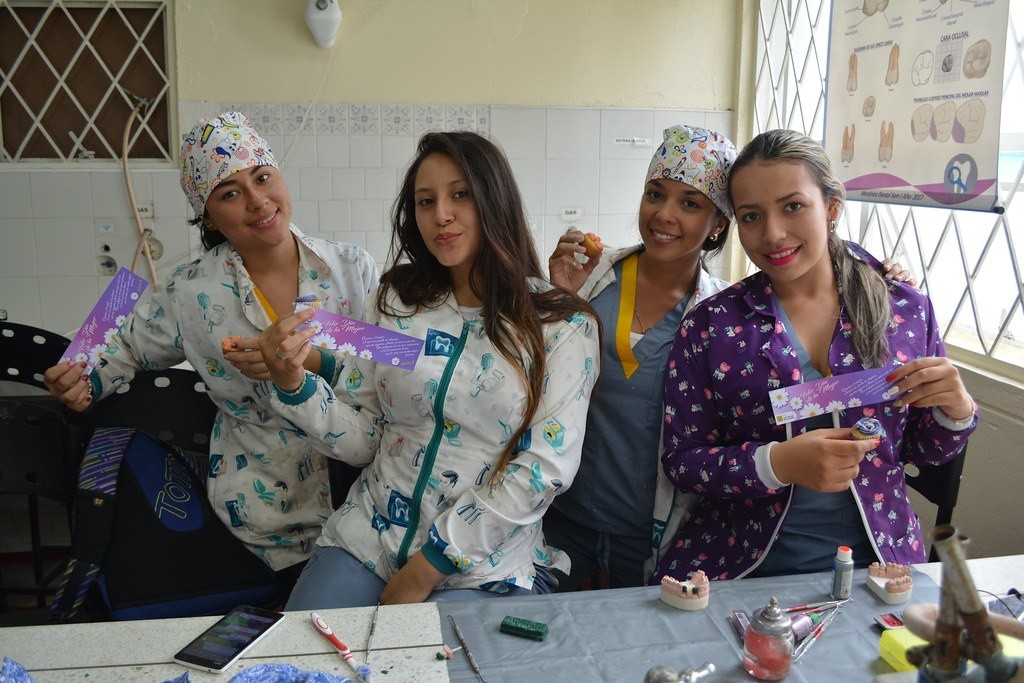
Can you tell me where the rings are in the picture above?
[276,346,290,360]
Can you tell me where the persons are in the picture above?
[648,129,979,587]
[540,126,738,595]
[43,112,379,611]
[257,131,601,609]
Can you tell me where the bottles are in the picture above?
[831,546,854,598]
[744,597,794,680]
[792,612,818,641]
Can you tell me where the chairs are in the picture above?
[1,320,217,609]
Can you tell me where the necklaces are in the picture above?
[634,306,647,334]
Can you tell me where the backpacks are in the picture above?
[30,426,254,625]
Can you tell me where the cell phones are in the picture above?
[173,605,285,674]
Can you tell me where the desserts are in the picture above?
[222,335,246,355]
[293,294,322,309]
[851,417,883,440]
[579,232,603,257]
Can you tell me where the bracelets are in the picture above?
[938,392,976,426]
[273,372,306,396]
[87,379,91,395]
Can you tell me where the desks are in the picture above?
[0,554,1024,683]
